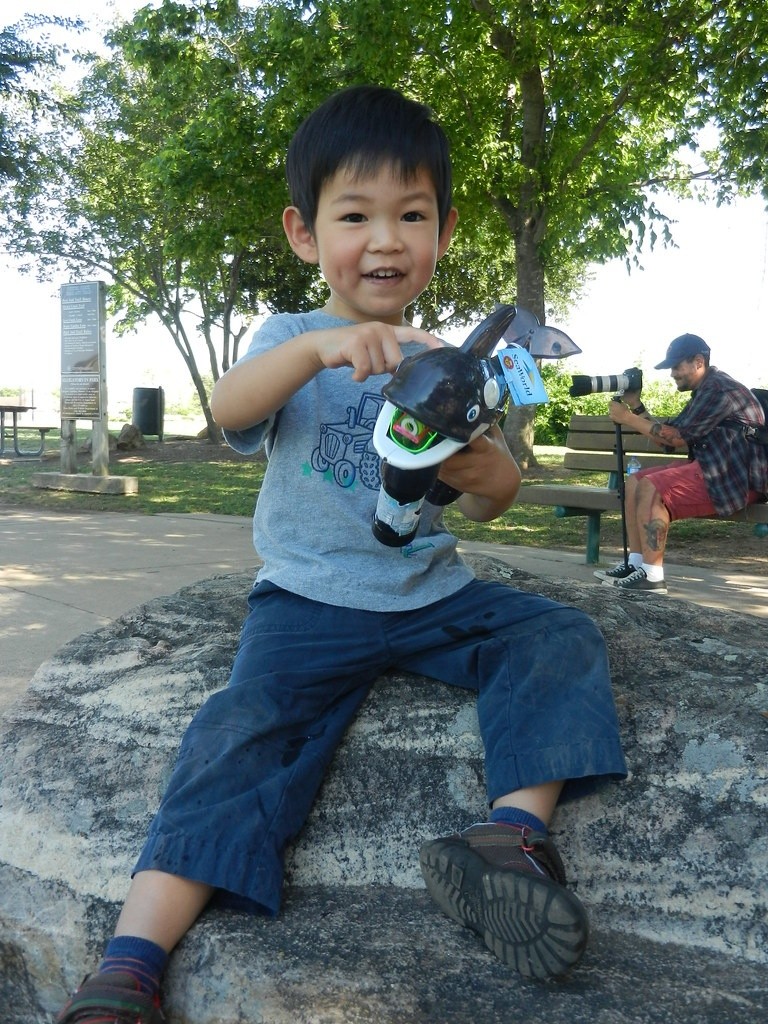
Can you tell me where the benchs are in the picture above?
[517,415,768,565]
[5,426,58,432]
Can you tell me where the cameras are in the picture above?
[569,367,644,397]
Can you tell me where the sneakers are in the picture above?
[54,970,165,1024]
[602,567,668,595]
[419,820,587,979]
[593,563,638,580]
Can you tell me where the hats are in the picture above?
[654,333,710,370]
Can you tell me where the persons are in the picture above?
[593,333,768,594]
[56,86,624,1023]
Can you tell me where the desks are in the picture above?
[0,405,37,456]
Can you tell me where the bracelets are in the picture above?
[633,403,645,415]
